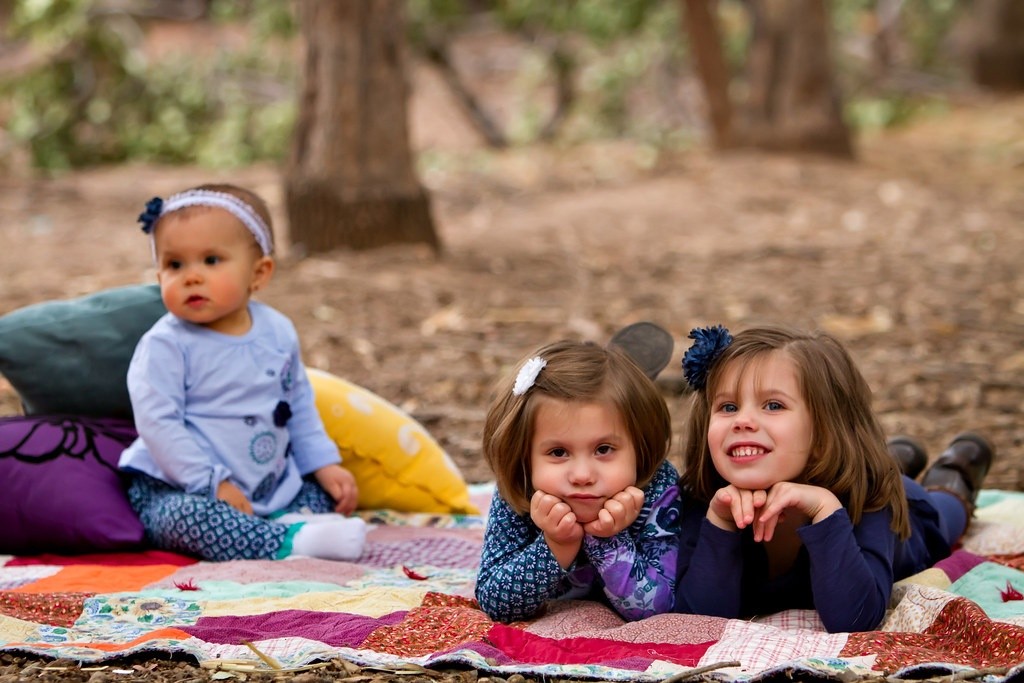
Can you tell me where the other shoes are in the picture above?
[607,321,673,379]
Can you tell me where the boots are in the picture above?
[920,429,994,535]
[887,436,928,482]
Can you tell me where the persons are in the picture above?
[111,183,370,561]
[473,320,687,624]
[672,325,997,633]
